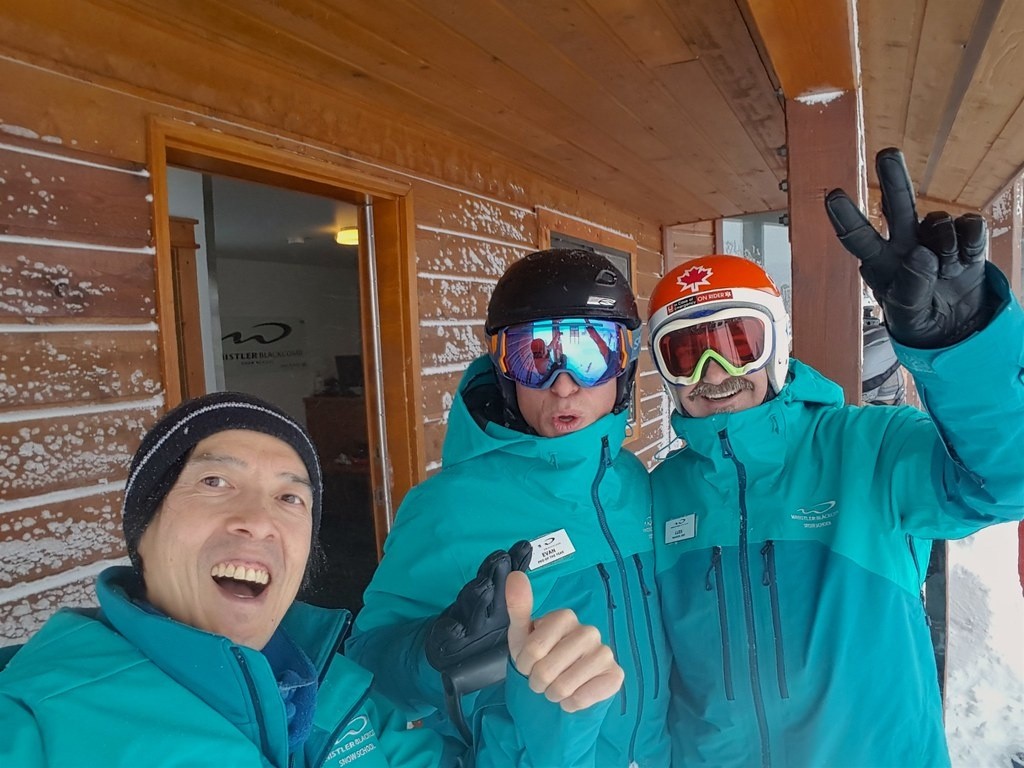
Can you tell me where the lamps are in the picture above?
[335,226,359,246]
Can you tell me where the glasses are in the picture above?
[648,307,781,384]
[484,318,650,389]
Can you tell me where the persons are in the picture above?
[344,248,674,768]
[648,146,1024,768]
[0,391,625,768]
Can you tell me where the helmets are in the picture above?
[646,254,793,392]
[481,250,646,332]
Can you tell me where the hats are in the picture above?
[115,387,323,566]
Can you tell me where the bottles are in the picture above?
[312,370,322,396]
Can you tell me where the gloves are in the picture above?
[821,146,990,350]
[420,542,536,674]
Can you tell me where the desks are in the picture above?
[302,396,368,465]
